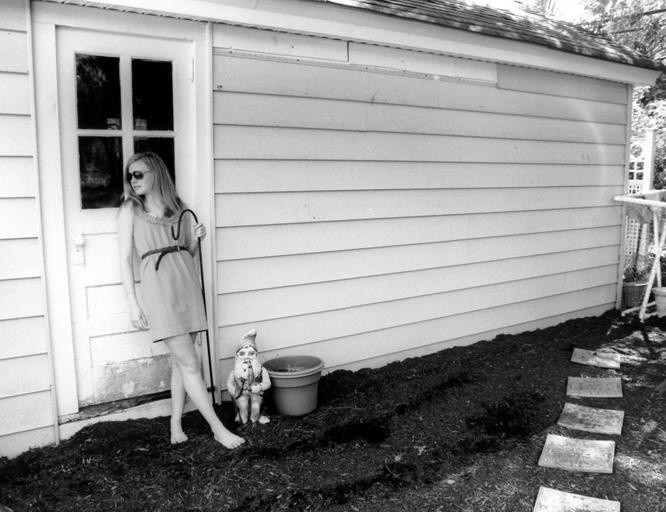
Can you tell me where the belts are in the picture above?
[141,245,186,271]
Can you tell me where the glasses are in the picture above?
[127,171,149,181]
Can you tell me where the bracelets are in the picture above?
[125,290,136,296]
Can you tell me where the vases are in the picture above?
[260,354,326,419]
[650,285,666,319]
[621,279,649,311]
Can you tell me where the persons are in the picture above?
[118,151,246,450]
[226,328,273,425]
